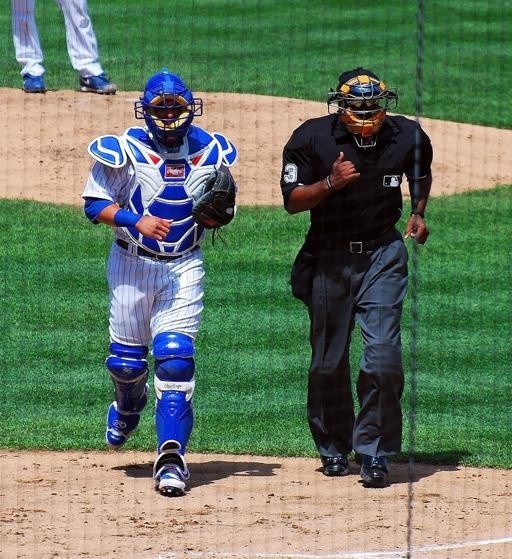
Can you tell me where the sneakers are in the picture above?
[80,73,117,94]
[102,383,150,447]
[22,73,47,92]
[154,468,188,494]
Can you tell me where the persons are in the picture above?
[279,67,434,486]
[10,0,117,94]
[80,70,240,497]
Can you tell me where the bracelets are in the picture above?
[113,204,142,230]
[325,170,337,193]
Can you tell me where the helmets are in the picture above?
[337,67,389,136]
[134,72,203,138]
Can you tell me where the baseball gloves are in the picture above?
[192,170,235,247]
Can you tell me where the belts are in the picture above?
[114,238,201,260]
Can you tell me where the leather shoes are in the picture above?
[354,449,389,487]
[320,454,351,477]
